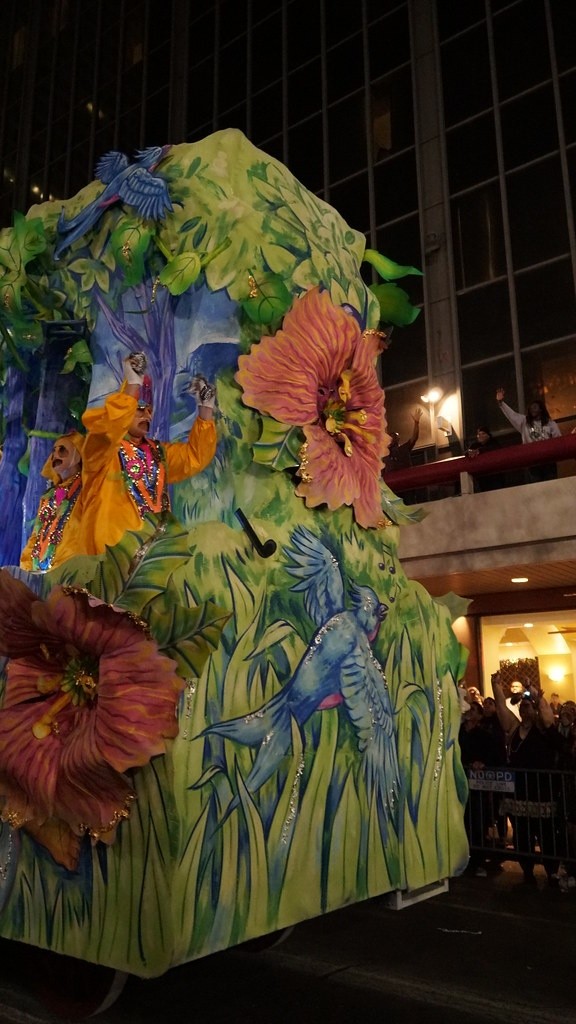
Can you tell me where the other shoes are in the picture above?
[543,875,559,887]
[522,873,537,884]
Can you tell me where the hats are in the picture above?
[477,427,492,438]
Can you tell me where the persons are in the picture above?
[463,424,512,492]
[495,386,563,482]
[458,671,576,899]
[382,408,423,505]
